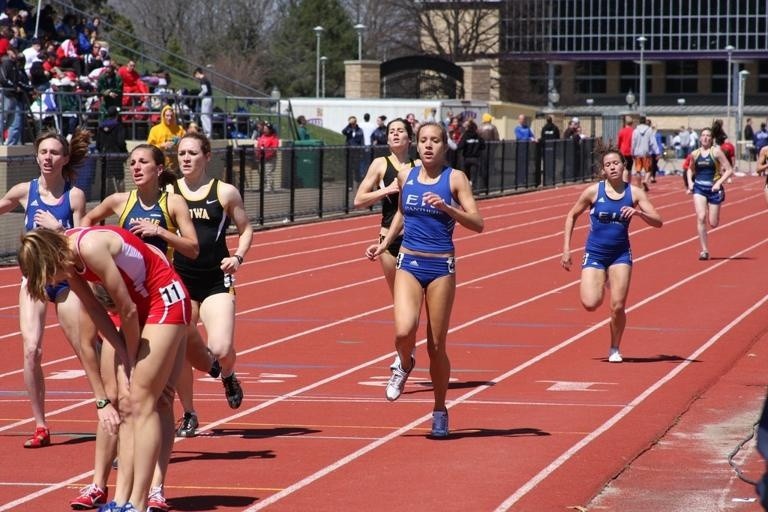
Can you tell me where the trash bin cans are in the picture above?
[281,140,325,188]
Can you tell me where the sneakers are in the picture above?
[697,248,710,262]
[23,426,54,449]
[606,345,624,362]
[427,407,451,439]
[145,494,174,509]
[178,412,200,439]
[69,486,110,510]
[384,351,416,403]
[99,498,139,512]
[218,369,244,410]
[204,346,221,380]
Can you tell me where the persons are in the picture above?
[0,130,254,512]
[561,147,664,361]
[343,108,501,188]
[512,114,587,180]
[354,118,484,437]
[0,0,309,191]
[615,108,768,260]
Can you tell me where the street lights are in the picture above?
[352,22,367,60]
[270,85,281,106]
[310,23,325,98]
[624,88,635,111]
[723,42,735,141]
[545,86,562,110]
[736,68,750,159]
[319,54,328,97]
[635,33,650,119]
